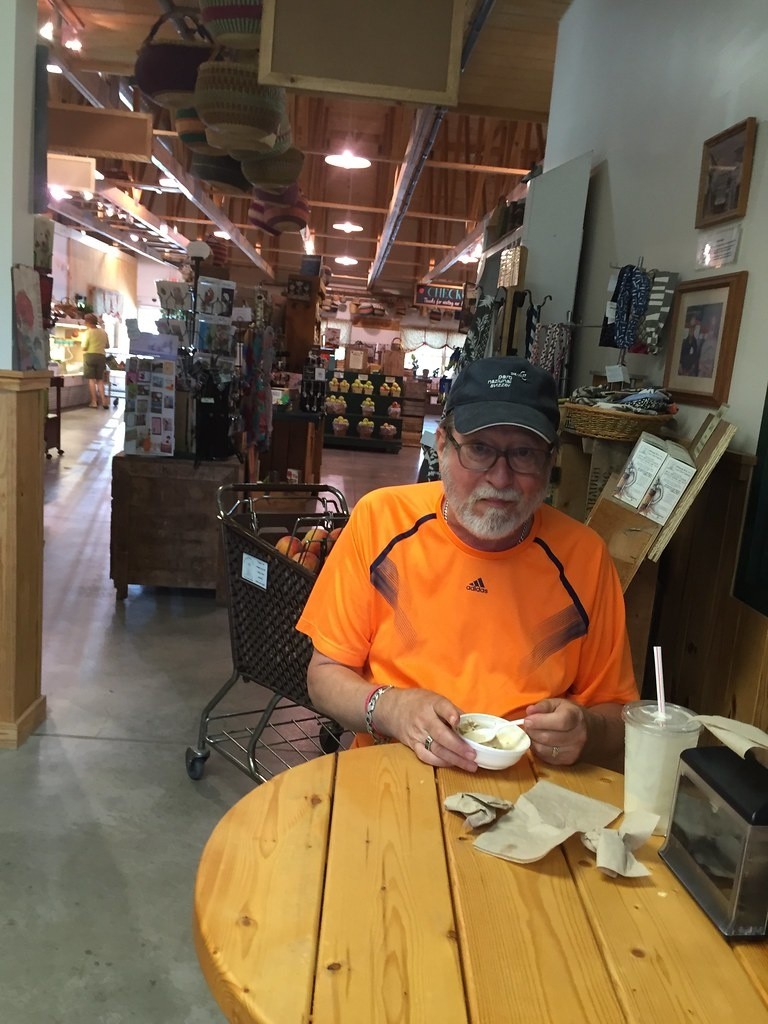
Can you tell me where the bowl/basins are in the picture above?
[452,713,532,771]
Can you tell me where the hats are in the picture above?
[440,356,561,444]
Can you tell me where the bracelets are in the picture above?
[365,684,395,745]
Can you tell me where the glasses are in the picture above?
[437,423,560,475]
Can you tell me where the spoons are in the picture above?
[463,718,524,743]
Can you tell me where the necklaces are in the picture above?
[444,500,529,545]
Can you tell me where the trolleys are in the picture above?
[184,482,359,786]
[101,367,126,409]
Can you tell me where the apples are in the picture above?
[325,378,401,428]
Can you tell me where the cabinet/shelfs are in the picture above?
[320,371,405,454]
[110,448,247,606]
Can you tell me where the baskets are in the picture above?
[562,401,674,442]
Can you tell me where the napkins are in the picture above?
[445,780,664,880]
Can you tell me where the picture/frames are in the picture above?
[695,114,758,228]
[257,0,466,106]
[660,268,749,408]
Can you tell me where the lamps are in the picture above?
[325,103,371,169]
[334,236,359,266]
[333,175,364,233]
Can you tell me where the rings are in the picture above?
[552,746,559,759]
[425,736,434,751]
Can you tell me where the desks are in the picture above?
[195,739,768,1024]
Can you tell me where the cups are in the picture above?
[620,700,702,836]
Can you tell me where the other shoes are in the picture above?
[88,404,97,408]
[103,405,109,409]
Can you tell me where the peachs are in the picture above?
[274,528,341,573]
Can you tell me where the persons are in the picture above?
[294,356,641,773]
[442,350,461,403]
[366,356,383,374]
[81,313,110,409]
